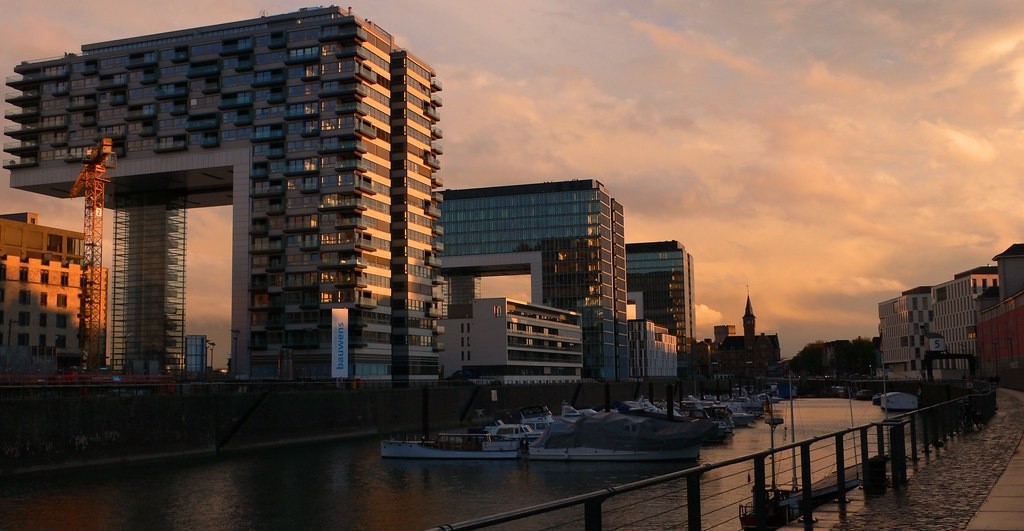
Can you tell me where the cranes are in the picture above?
[70,138,117,374]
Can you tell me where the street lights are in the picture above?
[208,342,216,370]
[7,319,21,347]
[231,329,240,375]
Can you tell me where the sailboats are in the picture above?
[739,393,803,531]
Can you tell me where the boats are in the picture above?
[856,390,873,400]
[434,383,797,445]
[379,432,521,458]
[872,393,883,405]
[529,413,719,459]
